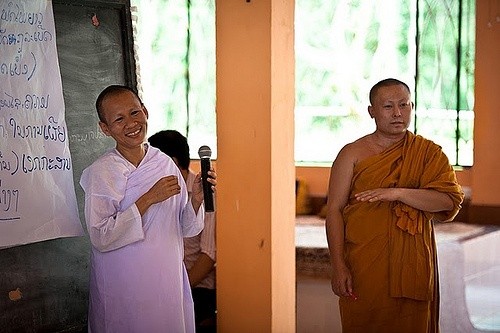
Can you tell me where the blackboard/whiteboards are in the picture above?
[0,0,146,333]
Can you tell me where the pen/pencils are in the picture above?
[347,291,357,300]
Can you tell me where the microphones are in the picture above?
[198,146,214,212]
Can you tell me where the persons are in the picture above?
[326,79,465,333]
[79,85,216,333]
[148,130,216,333]
